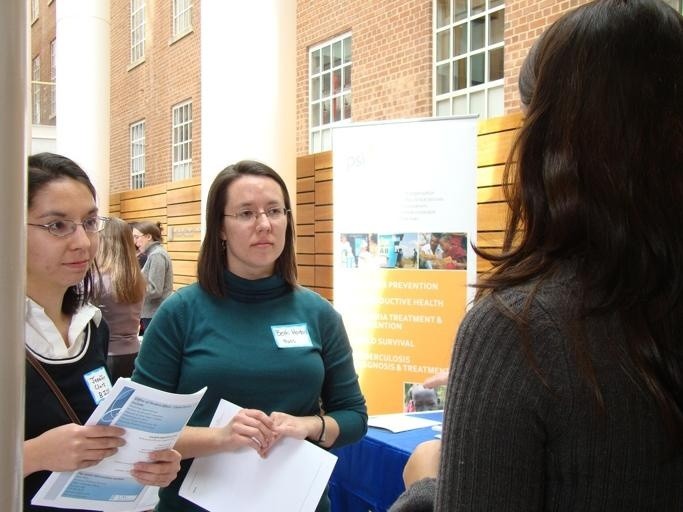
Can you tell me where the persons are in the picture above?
[394,247,404,268]
[358,239,370,266]
[72,217,146,387]
[430,236,467,269]
[132,220,174,334]
[382,1,683,511]
[341,235,352,265]
[129,222,148,269]
[411,248,417,267]
[23,151,182,512]
[420,233,443,269]
[369,235,378,256]
[130,157,371,512]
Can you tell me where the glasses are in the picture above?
[27,216,110,238]
[223,206,291,224]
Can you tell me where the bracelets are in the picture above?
[314,413,326,443]
